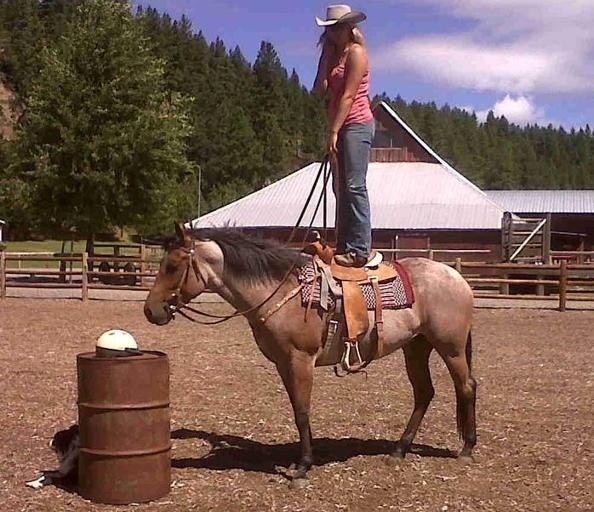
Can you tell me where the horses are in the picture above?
[145,220,477,479]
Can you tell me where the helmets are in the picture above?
[96,330,142,355]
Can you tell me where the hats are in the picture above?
[315,5,366,26]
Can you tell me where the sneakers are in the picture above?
[335,253,366,267]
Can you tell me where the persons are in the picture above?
[312,4,376,268]
